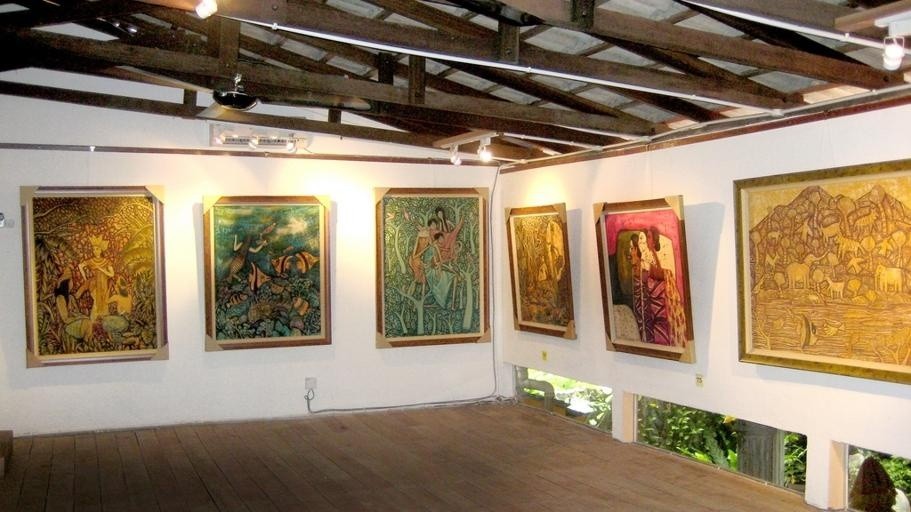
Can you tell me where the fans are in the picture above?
[115,65,371,118]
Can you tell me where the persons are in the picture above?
[626,234,685,347]
[100,275,132,335]
[233,233,269,258]
[635,227,661,268]
[53,264,92,343]
[409,206,468,311]
[78,232,113,320]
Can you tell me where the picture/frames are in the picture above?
[376,183,493,348]
[18,183,168,365]
[506,203,576,339]
[733,157,911,385]
[593,196,696,363]
[199,193,331,354]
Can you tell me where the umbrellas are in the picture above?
[848,455,900,511]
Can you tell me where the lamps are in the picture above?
[196,0,218,18]
[213,133,298,151]
[881,35,905,71]
[434,129,500,166]
